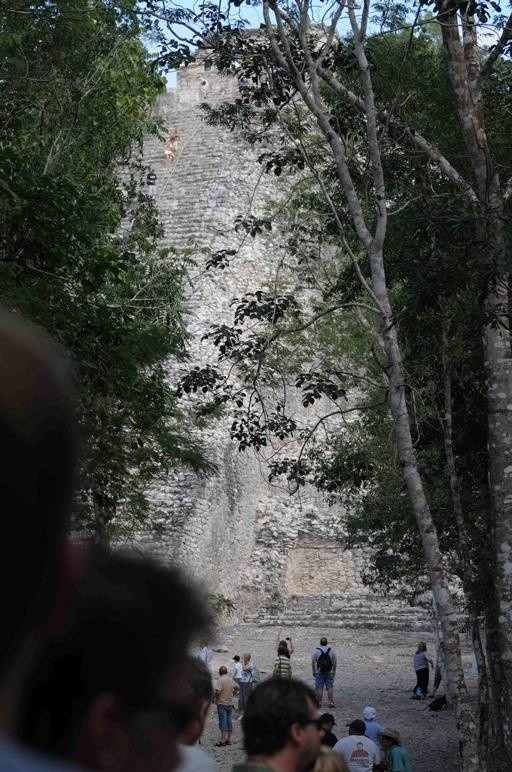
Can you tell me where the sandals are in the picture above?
[214,740,232,747]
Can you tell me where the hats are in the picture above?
[232,655,240,662]
[345,719,367,732]
[377,726,402,744]
[320,712,338,726]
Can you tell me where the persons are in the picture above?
[411,642,434,699]
[1,317,86,771]
[15,557,213,772]
[174,628,412,772]
[428,642,448,697]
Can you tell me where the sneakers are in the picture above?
[328,703,336,708]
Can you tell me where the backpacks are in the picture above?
[315,646,335,674]
